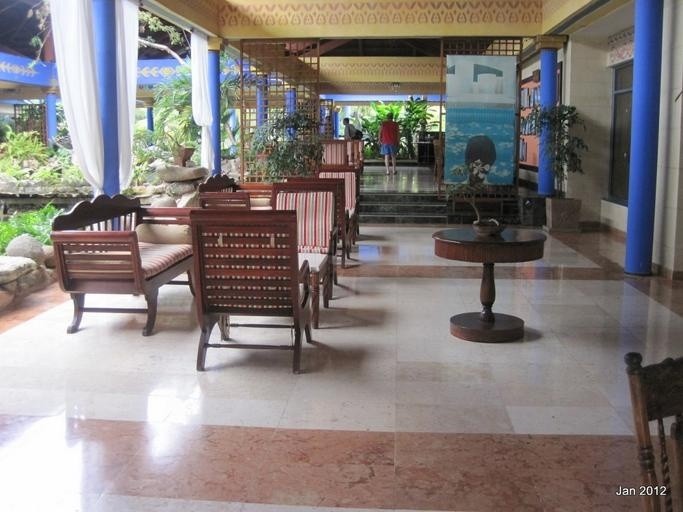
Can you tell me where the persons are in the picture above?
[379,112,400,175]
[323,115,334,139]
[343,118,356,141]
[465,135,496,184]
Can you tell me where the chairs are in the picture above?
[197,173,273,211]
[347,139,354,165]
[315,164,360,245]
[283,176,351,271]
[189,208,305,375]
[49,193,189,336]
[321,140,347,166]
[623,352,683,512]
[354,140,360,165]
[360,140,364,163]
[271,181,342,300]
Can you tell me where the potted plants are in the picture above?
[515,102,587,235]
[444,160,506,237]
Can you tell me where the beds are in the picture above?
[432,228,547,344]
